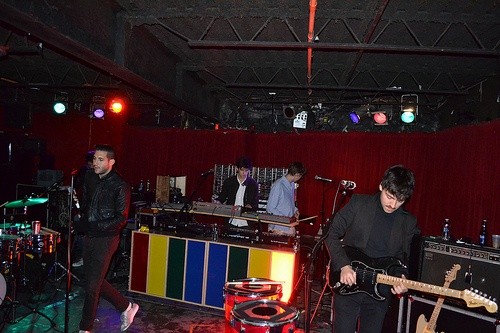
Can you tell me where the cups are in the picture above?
[492,235,500,248]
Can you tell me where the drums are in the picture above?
[229,299,302,333]
[0,223,31,237]
[223,277,283,325]
[20,229,57,255]
[0,271,8,307]
[0,234,24,266]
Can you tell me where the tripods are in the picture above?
[2,276,56,327]
[45,236,81,282]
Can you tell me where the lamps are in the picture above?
[282,104,303,120]
[50,88,71,116]
[365,99,398,128]
[108,95,129,114]
[400,92,420,128]
[350,102,371,124]
[88,94,108,121]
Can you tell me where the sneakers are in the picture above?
[120,302,139,331]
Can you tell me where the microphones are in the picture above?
[315,175,332,182]
[464,265,473,284]
[200,169,214,176]
[341,180,357,189]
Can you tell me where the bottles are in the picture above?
[480,220,488,245]
[139,179,143,190]
[146,179,150,191]
[441,218,451,241]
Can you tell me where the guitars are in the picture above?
[415,263,462,333]
[328,245,498,313]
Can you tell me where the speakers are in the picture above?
[419,236,500,318]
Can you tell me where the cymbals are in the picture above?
[5,198,49,208]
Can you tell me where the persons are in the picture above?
[327,167,417,333]
[216,159,259,229]
[266,161,305,235]
[70,144,139,333]
